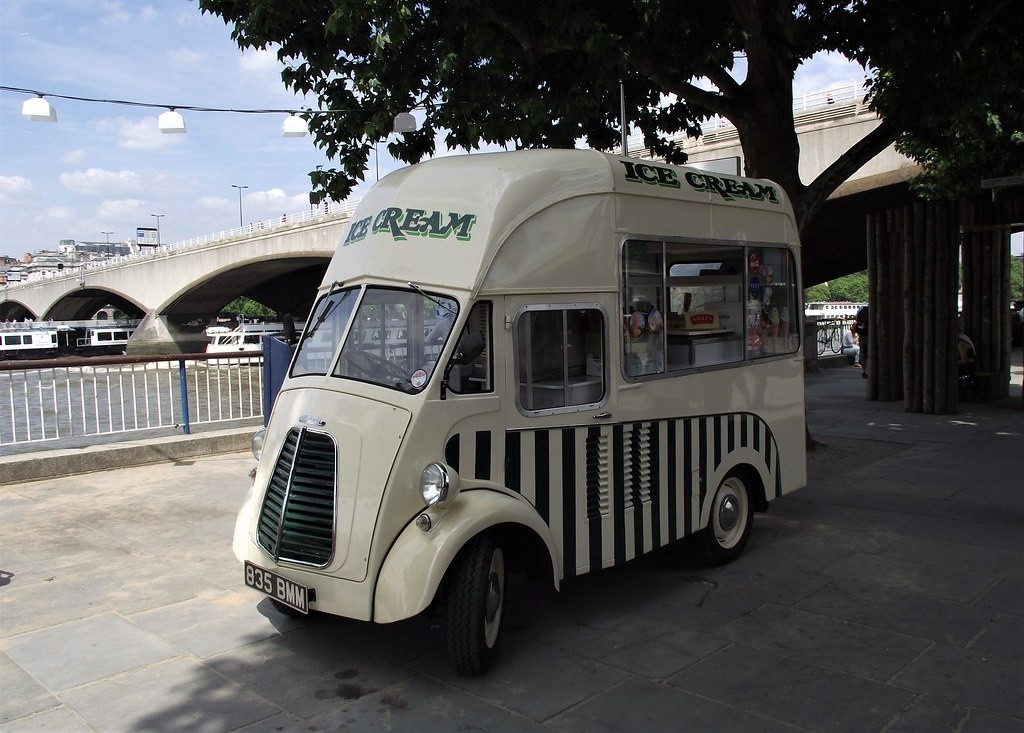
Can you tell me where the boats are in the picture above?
[2,322,70,356]
[79,322,133,360]
[201,319,291,367]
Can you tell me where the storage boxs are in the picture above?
[585,354,602,376]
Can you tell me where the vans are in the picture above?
[233,154,807,675]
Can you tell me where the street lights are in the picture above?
[151,214,165,244]
[230,183,247,225]
[102,229,112,254]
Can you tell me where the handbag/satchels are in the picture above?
[851,323,868,335]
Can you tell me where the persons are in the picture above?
[248,222,253,232]
[0,318,33,329]
[323,199,328,214]
[856,300,870,378]
[827,94,834,104]
[842,323,861,367]
[49,316,53,326]
[631,297,664,372]
[281,214,286,225]
[258,220,264,229]
[425,300,469,345]
[956,329,981,375]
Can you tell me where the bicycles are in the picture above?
[820,316,851,355]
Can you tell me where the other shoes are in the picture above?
[862,373,868,379]
